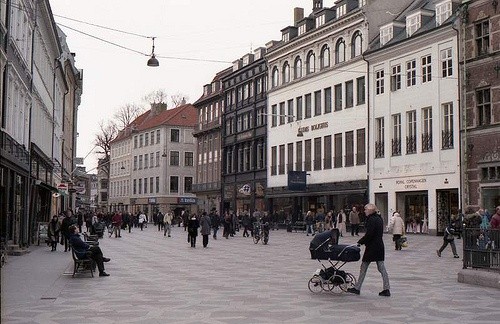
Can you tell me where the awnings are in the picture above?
[479,182,500,189]
[37,180,59,193]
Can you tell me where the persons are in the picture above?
[436,225,459,258]
[112,211,122,238]
[317,211,325,221]
[325,208,346,237]
[347,203,391,296]
[69,225,110,276]
[188,214,199,247]
[154,209,175,237]
[349,207,360,236]
[456,206,500,268]
[390,212,405,250]
[97,208,148,233]
[306,210,315,236]
[201,212,211,247]
[177,209,278,240]
[260,212,270,245]
[48,208,84,252]
[85,211,94,232]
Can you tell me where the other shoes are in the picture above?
[379,289,390,296]
[312,234,315,236]
[55,249,56,251]
[65,249,67,252]
[51,250,53,251]
[102,257,110,262]
[454,255,459,258]
[99,272,110,276]
[395,247,402,250]
[69,247,71,251]
[307,234,308,236]
[457,238,461,239]
[438,250,441,257]
[347,288,360,294]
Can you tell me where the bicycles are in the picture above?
[253,222,268,245]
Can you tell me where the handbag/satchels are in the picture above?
[400,233,408,247]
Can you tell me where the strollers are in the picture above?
[307,228,363,295]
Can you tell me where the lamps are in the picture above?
[162,145,167,157]
[120,159,126,170]
[294,116,304,136]
[49,190,61,198]
[144,35,160,68]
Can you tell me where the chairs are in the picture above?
[68,223,106,278]
